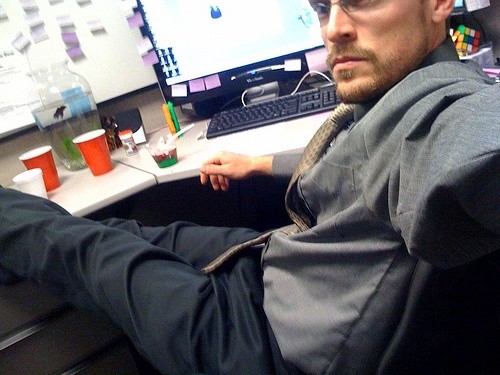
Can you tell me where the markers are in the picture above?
[161,103,179,140]
[168,101,182,138]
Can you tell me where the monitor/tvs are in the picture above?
[136,0,325,111]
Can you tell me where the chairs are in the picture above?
[374,243,467,370]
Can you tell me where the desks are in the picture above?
[106,71,357,186]
[1,155,158,372]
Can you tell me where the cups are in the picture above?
[145,134,178,168]
[72,129,113,176]
[18,145,61,192]
[12,168,48,201]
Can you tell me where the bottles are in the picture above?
[118,129,139,157]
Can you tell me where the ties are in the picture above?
[199,102,359,273]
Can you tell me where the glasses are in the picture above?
[298,0,374,28]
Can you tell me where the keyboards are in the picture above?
[206,85,343,138]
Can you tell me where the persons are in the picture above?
[0,0,500,373]
